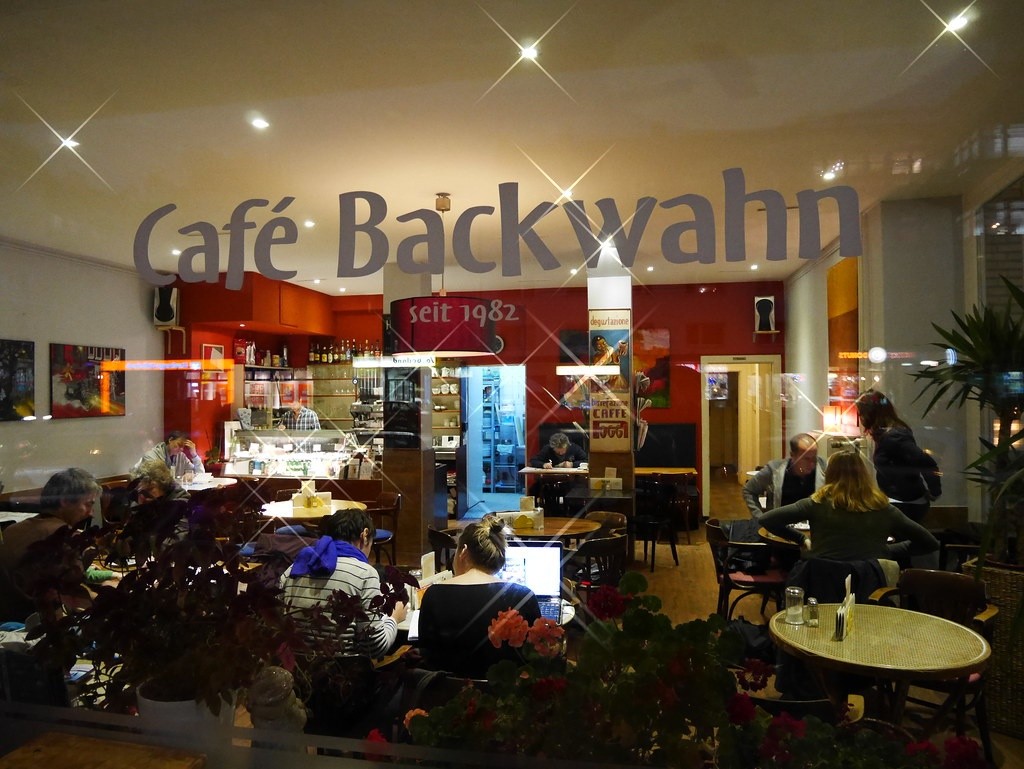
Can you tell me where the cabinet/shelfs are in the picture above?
[434,374,463,430]
[231,361,358,427]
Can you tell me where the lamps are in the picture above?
[390,193,496,359]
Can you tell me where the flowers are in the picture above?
[405,571,986,769]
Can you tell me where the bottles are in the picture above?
[255,345,288,367]
[601,479,611,493]
[784,586,804,624]
[308,338,381,365]
[807,596,819,628]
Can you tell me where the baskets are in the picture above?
[960,556,1024,739]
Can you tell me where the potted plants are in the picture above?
[908,273,1024,742]
[25,469,422,752]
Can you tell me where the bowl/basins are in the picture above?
[435,400,460,411]
[432,383,459,394]
[443,412,460,427]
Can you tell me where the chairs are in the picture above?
[0,463,1024,769]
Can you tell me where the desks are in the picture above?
[181,477,237,493]
[767,602,991,725]
[87,555,263,604]
[510,517,603,562]
[519,467,588,507]
[635,467,698,543]
[0,732,210,769]
[759,525,813,554]
[255,498,368,528]
[0,511,38,527]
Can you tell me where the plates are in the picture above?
[794,524,810,530]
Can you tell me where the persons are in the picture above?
[278,389,321,453]
[417,514,542,678]
[856,390,942,570]
[0,466,126,626]
[742,434,827,520]
[528,433,588,518]
[129,458,190,513]
[277,508,408,713]
[141,431,205,476]
[757,450,939,562]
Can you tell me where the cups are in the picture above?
[185,469,194,485]
[431,367,461,378]
[302,481,316,506]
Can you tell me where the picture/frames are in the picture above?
[200,343,224,373]
